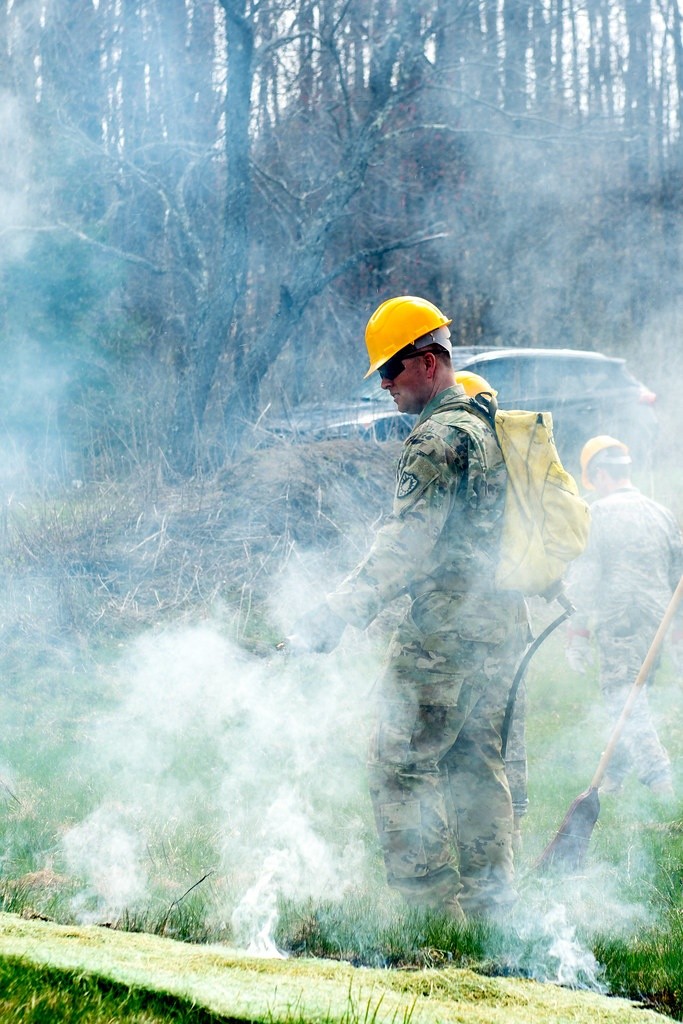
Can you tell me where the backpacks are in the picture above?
[414,392,591,598]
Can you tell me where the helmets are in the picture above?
[362,295,454,380]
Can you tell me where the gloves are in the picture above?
[565,628,594,687]
[671,627,683,687]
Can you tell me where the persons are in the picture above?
[556,433,681,835]
[297,293,539,971]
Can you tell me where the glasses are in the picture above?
[377,350,449,380]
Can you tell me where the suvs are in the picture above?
[257,341,658,481]
